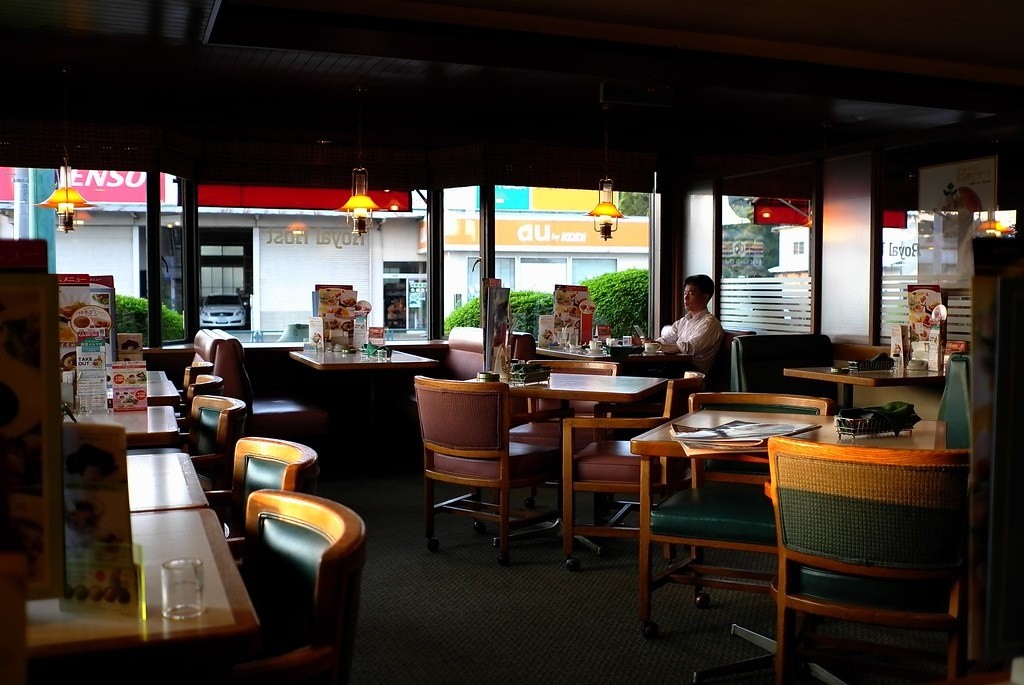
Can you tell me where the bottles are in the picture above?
[593,335,599,341]
[510,358,519,372]
[893,353,901,369]
[325,340,333,348]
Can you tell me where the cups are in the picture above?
[569,333,581,353]
[162,558,206,621]
[589,340,602,350]
[75,395,93,417]
[644,342,662,352]
[361,349,369,361]
[623,336,633,346]
[377,350,388,361]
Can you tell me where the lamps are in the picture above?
[334,85,391,236]
[33,65,99,234]
[580,105,632,241]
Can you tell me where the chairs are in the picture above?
[177,373,225,436]
[177,360,213,408]
[182,394,246,461]
[765,435,972,685]
[390,375,562,564]
[608,370,706,508]
[205,436,321,541]
[222,489,369,685]
[509,359,620,454]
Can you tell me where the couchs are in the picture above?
[731,333,850,416]
[382,326,536,406]
[190,328,329,441]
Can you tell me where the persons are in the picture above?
[611,274,725,440]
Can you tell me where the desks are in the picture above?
[630,410,947,638]
[466,372,668,526]
[106,381,182,409]
[146,371,167,382]
[289,350,438,406]
[143,346,304,396]
[783,366,945,410]
[26,507,261,659]
[126,452,210,512]
[64,405,180,445]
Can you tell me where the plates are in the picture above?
[73,316,90,328]
[585,348,604,353]
[64,584,131,605]
[114,391,139,407]
[643,351,662,355]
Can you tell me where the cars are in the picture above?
[199,292,248,330]
